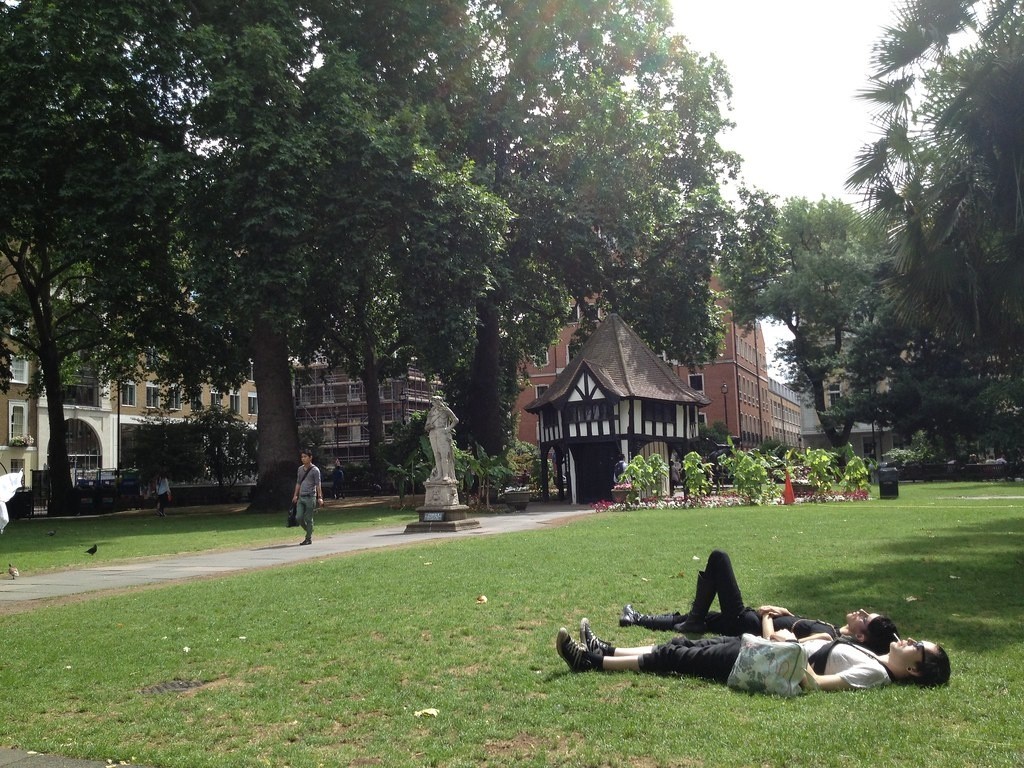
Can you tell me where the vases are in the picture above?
[505,491,530,510]
[611,490,631,503]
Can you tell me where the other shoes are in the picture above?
[163,514,166,517]
[300,537,311,544]
[158,511,162,516]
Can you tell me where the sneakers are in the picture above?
[580,617,612,657]
[556,628,586,674]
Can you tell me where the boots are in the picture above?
[674,571,718,633]
[619,605,680,629]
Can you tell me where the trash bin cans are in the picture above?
[878,467,899,500]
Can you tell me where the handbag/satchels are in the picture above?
[728,633,822,697]
[168,495,173,501]
[286,503,299,527]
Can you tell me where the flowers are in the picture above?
[613,483,632,490]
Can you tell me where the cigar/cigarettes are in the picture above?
[893,632,900,644]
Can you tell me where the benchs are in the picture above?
[899,464,1008,482]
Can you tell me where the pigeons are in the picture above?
[9,564,19,578]
[84,544,99,555]
[45,530,56,536]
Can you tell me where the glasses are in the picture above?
[914,641,927,674]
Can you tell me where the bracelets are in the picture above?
[319,496,322,498]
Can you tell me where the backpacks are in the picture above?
[614,461,624,479]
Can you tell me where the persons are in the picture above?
[425,394,459,478]
[555,616,951,692]
[614,454,629,483]
[333,465,345,499]
[520,469,530,486]
[620,551,900,655]
[292,451,324,545]
[150,467,171,516]
[968,451,1008,465]
[667,456,682,486]
